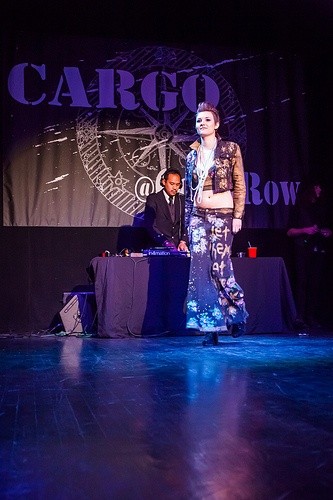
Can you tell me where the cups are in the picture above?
[248,247,257,258]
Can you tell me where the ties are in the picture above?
[169,197,174,207]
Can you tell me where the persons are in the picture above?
[183,103,249,347]
[287,179,333,328]
[145,167,190,253]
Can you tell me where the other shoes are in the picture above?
[202,331,218,346]
[232,322,245,337]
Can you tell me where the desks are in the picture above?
[90,257,298,340]
